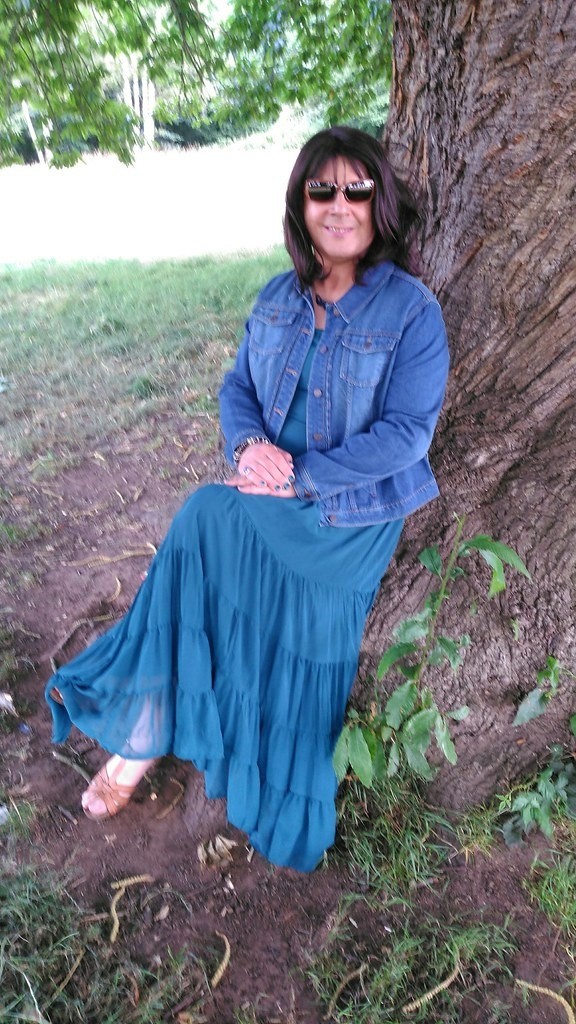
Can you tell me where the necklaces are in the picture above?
[314,291,328,311]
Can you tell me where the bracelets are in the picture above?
[233,436,272,468]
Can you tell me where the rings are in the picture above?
[243,469,249,477]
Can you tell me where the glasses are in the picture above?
[306,179,376,203]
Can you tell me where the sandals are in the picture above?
[81,750,164,821]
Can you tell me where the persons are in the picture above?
[49,127,451,881]
